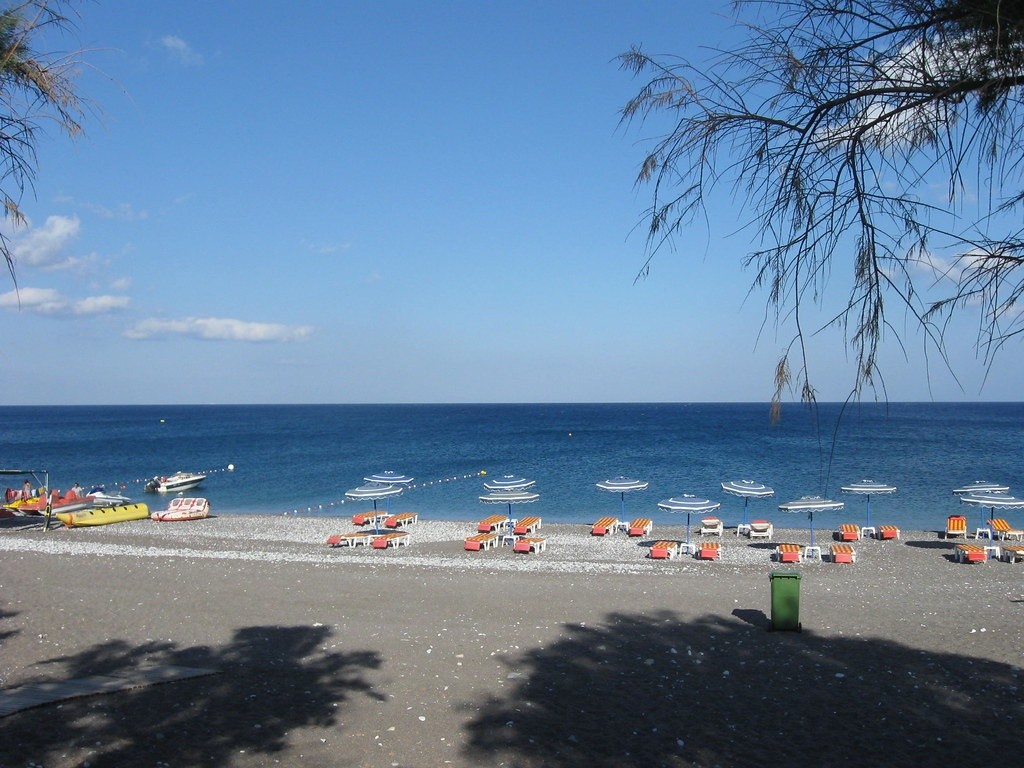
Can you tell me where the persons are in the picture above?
[71,483,84,497]
[22,481,34,491]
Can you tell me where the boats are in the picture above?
[2,487,95,518]
[155,470,207,493]
[86,486,131,507]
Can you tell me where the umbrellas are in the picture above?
[960,491,1024,560]
[841,479,897,537]
[483,474,537,519]
[952,480,1010,539]
[478,491,539,544]
[363,470,415,515]
[657,494,720,556]
[778,496,844,560]
[595,475,649,535]
[720,479,775,536]
[344,482,403,542]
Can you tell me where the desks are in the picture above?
[379,514,393,523]
[861,527,877,539]
[503,519,518,532]
[983,545,1001,561]
[679,542,696,557]
[805,546,821,561]
[737,524,751,537]
[502,536,519,548]
[615,522,631,533]
[975,528,991,540]
[366,535,384,545]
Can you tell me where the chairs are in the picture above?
[353,510,388,527]
[698,543,721,560]
[829,544,856,563]
[700,518,723,537]
[372,532,410,550]
[1002,546,1024,564]
[954,544,987,564]
[513,517,542,535]
[592,517,618,536]
[477,514,508,532]
[326,533,372,548]
[515,537,547,554]
[464,533,499,552]
[648,541,677,559]
[776,544,802,564]
[11,488,77,507]
[386,512,419,530]
[987,519,1024,543]
[838,524,860,541]
[629,519,652,538]
[878,525,900,540]
[944,515,967,540]
[750,521,774,540]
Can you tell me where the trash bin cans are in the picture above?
[766,571,802,634]
